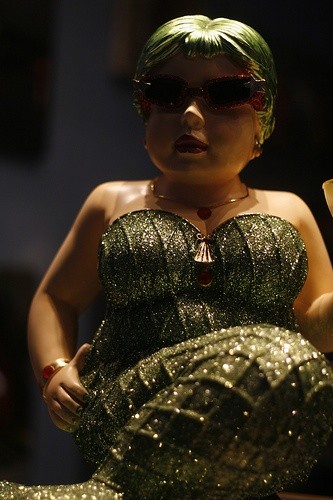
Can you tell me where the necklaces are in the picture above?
[147,174,250,221]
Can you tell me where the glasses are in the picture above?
[132,74,265,112]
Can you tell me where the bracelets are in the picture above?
[37,358,68,401]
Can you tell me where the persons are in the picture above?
[1,21,333,500]
